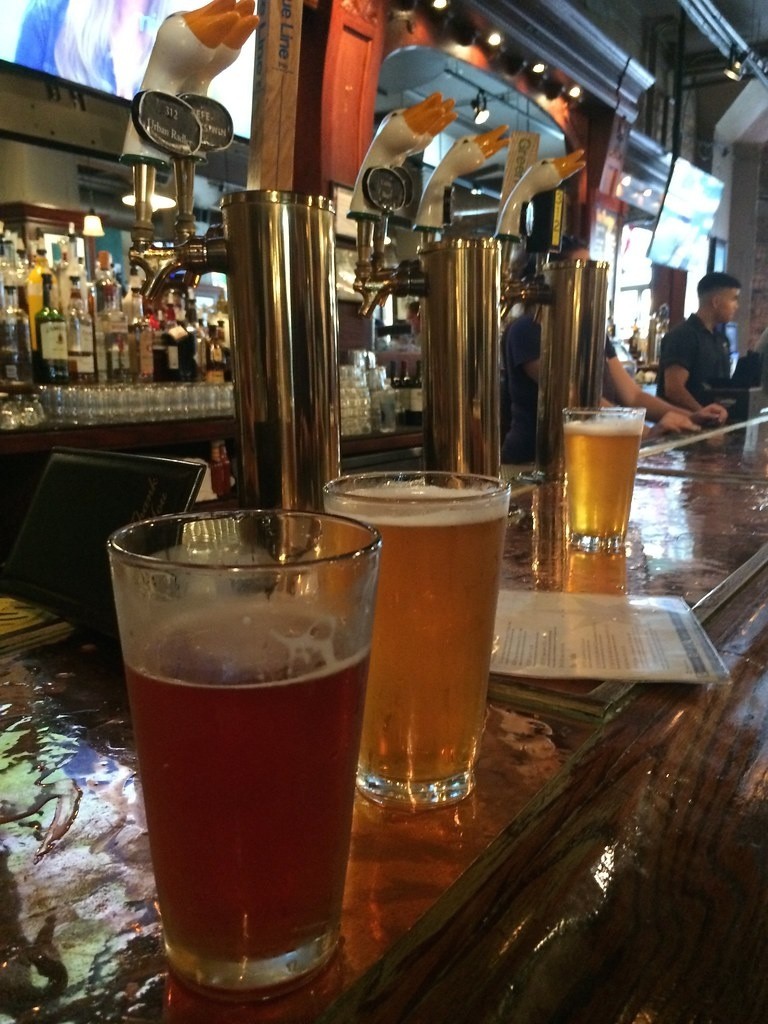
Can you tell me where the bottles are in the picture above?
[159,286,206,381]
[207,326,225,383]
[68,276,96,385]
[410,360,422,427]
[401,361,411,423]
[61,232,89,315]
[34,274,70,386]
[94,285,130,385]
[0,287,32,383]
[28,248,58,352]
[390,360,401,424]
[129,294,155,384]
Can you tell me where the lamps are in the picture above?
[82,186,106,238]
[723,44,749,81]
[470,89,490,125]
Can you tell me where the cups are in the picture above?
[561,407,646,552]
[106,509,383,1000]
[326,471,512,813]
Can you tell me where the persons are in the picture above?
[730,326,768,388]
[500,302,729,466]
[398,302,422,382]
[659,274,741,412]
